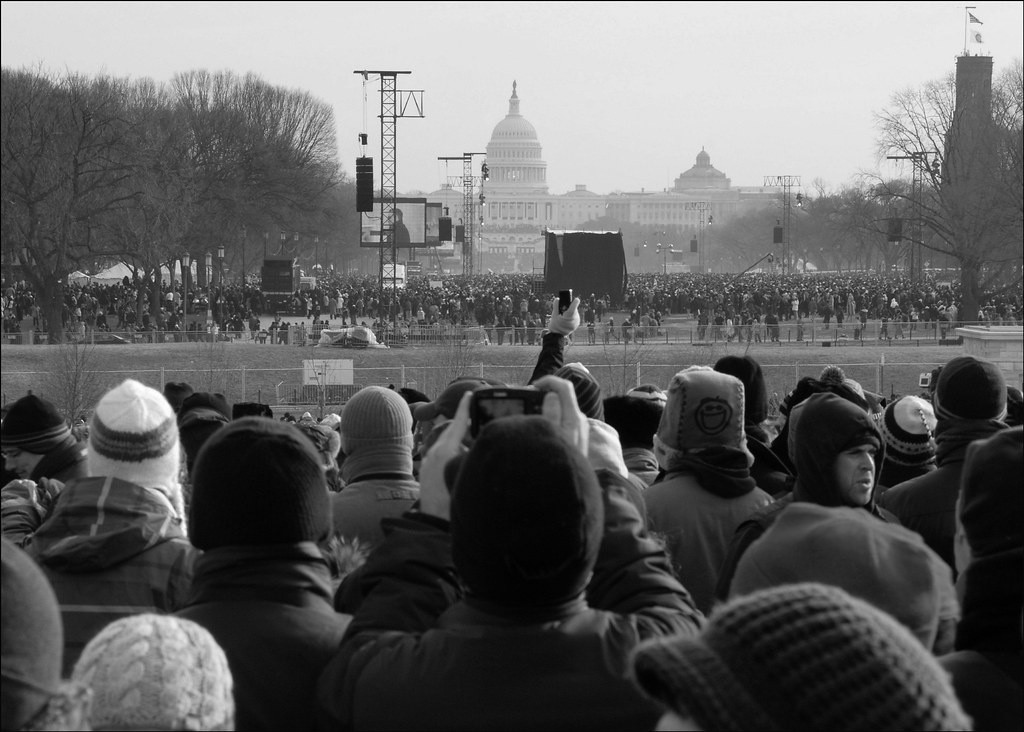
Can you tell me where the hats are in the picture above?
[627,581,972,732]
[88,378,182,490]
[933,357,1007,426]
[2,390,77,456]
[1008,384,1024,415]
[957,424,1024,557]
[651,363,755,469]
[186,416,334,551]
[447,414,603,604]
[71,611,236,732]
[774,366,937,466]
[162,360,666,491]
[0,535,64,732]
[724,503,961,652]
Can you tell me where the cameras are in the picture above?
[470,387,544,435]
[919,373,932,387]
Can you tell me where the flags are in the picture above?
[968,11,988,54]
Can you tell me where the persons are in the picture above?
[0,297,1024,732]
[383,208,411,244]
[0,271,1024,346]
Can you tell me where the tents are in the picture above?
[65,258,213,286]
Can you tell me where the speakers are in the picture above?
[439,217,452,242]
[774,227,783,243]
[690,240,698,252]
[356,157,374,213]
[634,248,640,256]
[888,217,902,242]
[456,225,465,242]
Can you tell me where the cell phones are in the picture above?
[558,289,572,314]
